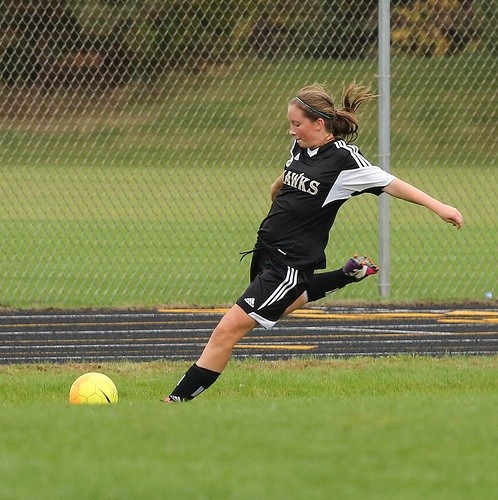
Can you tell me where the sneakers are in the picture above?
[343,254,379,279]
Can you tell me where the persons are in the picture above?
[159,82,462,404]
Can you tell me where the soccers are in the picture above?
[68,372,119,405]
